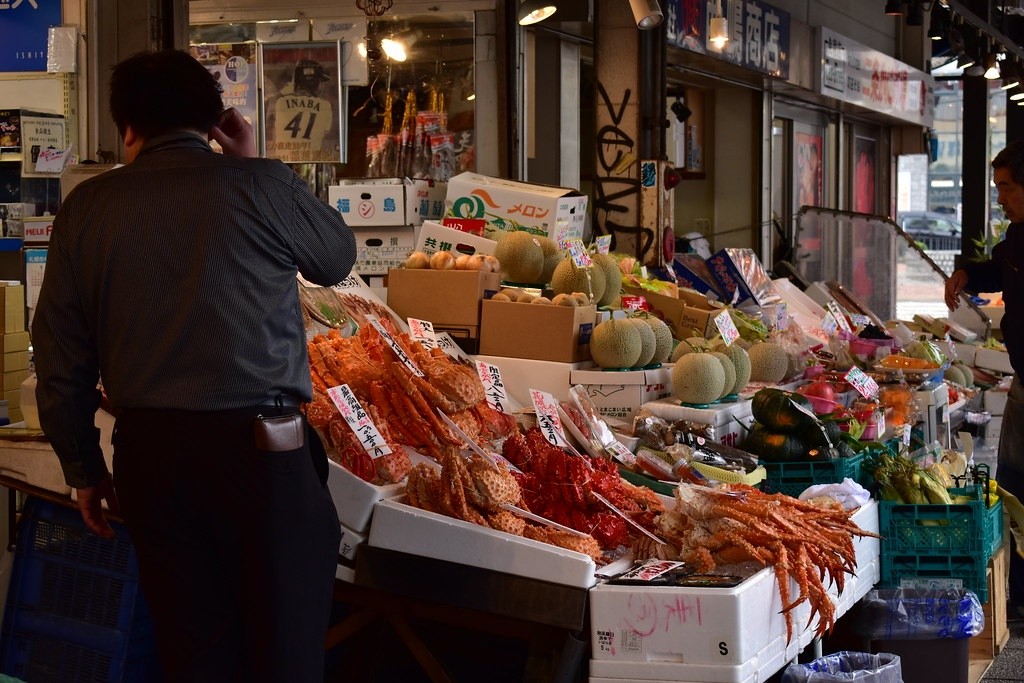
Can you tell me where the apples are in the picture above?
[836,409,875,426]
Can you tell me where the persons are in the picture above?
[35,51,356,683]
[797,142,820,240]
[262,59,334,160]
[944,138,1024,622]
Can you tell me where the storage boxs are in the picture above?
[0,171,1014,683]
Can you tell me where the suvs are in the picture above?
[897,211,962,250]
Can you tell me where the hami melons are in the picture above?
[588,306,788,404]
[942,363,974,388]
[482,219,564,286]
[551,245,623,306]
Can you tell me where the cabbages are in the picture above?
[907,339,946,365]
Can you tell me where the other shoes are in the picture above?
[1007,600,1024,622]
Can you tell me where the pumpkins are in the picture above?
[746,387,855,482]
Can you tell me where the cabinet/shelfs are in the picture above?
[0,72,78,285]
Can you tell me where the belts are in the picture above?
[259,397,298,407]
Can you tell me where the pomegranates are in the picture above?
[403,251,501,272]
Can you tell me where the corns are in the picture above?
[874,451,965,548]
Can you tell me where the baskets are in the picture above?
[762,432,903,498]
[878,485,1003,604]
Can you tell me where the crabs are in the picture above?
[302,316,885,648]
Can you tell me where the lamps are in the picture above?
[956,51,975,69]
[381,21,423,63]
[1001,72,1019,90]
[927,5,947,40]
[671,95,693,123]
[630,0,664,30]
[885,0,904,16]
[966,56,985,76]
[1009,85,1024,100]
[984,64,1000,79]
[710,0,729,48]
[518,0,557,26]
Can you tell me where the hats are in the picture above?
[294,59,331,84]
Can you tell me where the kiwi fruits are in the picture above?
[491,289,590,306]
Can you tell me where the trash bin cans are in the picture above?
[784,653,902,683]
[866,586,973,683]
